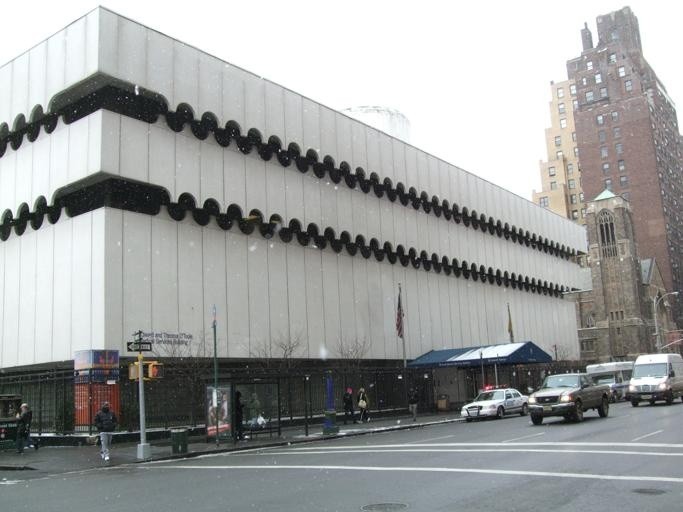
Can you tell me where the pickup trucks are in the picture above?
[527,372,611,425]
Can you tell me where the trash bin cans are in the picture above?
[437,394,450,412]
[171,426,190,454]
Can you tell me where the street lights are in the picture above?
[650,292,679,354]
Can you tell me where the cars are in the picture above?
[460,384,530,422]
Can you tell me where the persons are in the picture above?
[235,390,247,441]
[356,387,371,423]
[14,402,33,455]
[92,401,119,462]
[247,392,264,419]
[407,384,419,422]
[343,387,360,425]
[207,395,216,426]
[220,392,227,425]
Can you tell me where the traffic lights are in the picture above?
[129,362,139,381]
[149,364,165,378]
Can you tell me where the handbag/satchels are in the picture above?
[358,400,367,409]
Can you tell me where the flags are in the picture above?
[396,291,405,340]
[508,306,514,341]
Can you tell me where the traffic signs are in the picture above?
[127,341,152,352]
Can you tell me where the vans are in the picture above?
[629,353,682,407]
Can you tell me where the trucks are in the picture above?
[586,361,634,403]
